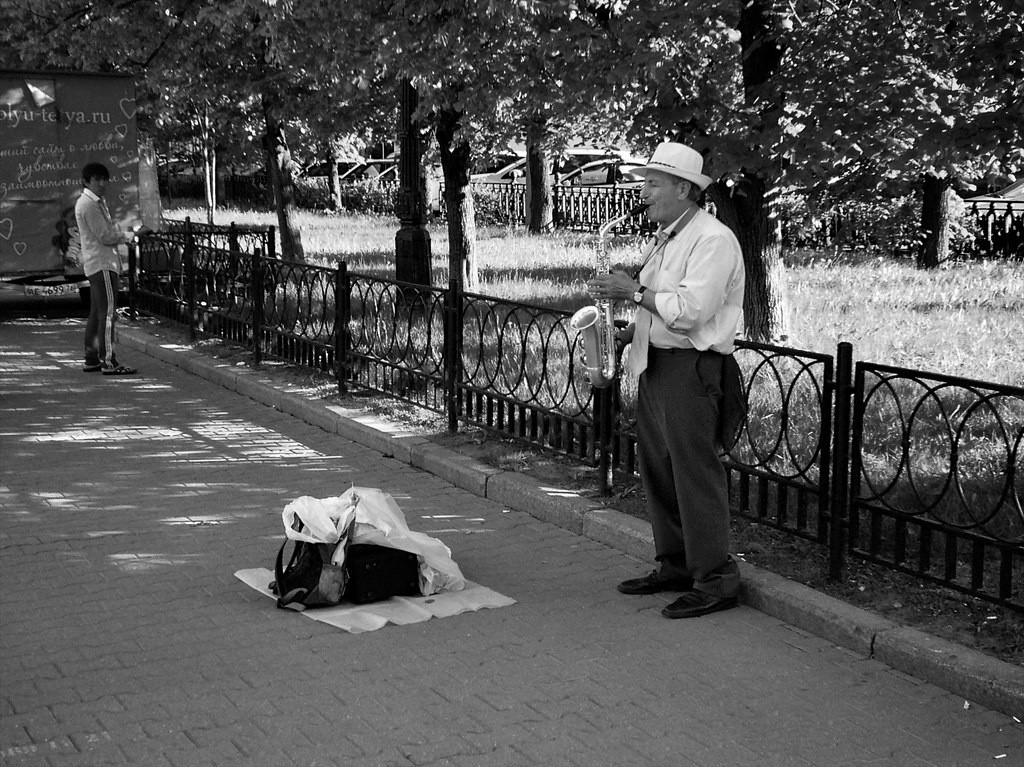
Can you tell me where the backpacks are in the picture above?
[268,496,440,612]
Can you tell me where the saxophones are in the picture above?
[570,202,654,389]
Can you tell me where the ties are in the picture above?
[627,232,669,378]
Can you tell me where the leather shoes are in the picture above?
[617,568,692,595]
[661,587,743,620]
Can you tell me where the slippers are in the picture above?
[82,362,102,372]
[100,365,137,375]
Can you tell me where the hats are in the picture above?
[629,141,713,191]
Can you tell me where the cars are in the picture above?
[293,147,647,194]
[962,177,1024,228]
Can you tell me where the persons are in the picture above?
[587,141,745,618]
[74,162,137,375]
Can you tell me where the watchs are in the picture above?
[633,286,647,305]
